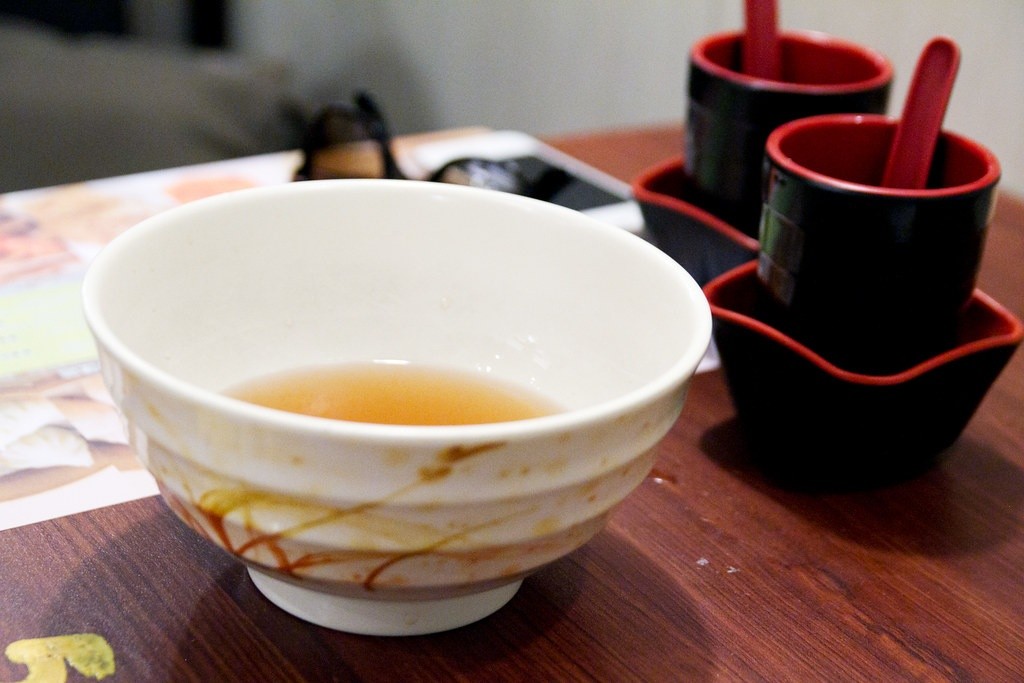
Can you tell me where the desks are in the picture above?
[0,125,1024,683]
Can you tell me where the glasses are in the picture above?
[290,90,578,201]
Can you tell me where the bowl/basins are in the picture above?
[81,178,713,637]
[701,258,1024,492]
[636,155,760,287]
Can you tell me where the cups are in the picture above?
[755,114,1004,365]
[682,29,897,231]
